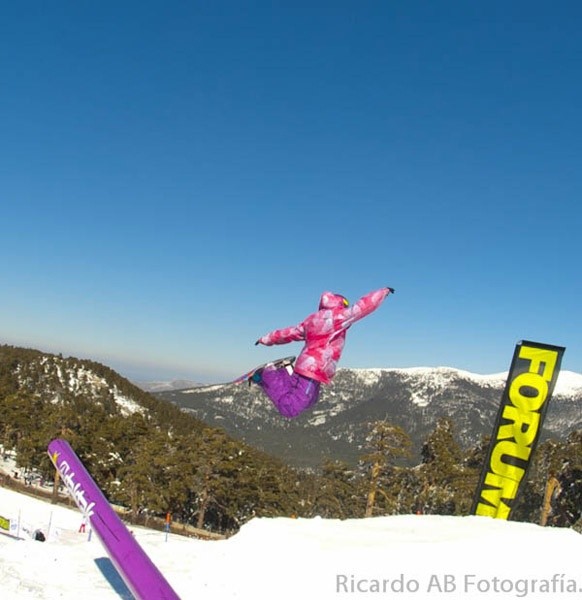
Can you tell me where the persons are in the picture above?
[250,287,395,418]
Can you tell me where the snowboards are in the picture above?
[232,356,298,385]
